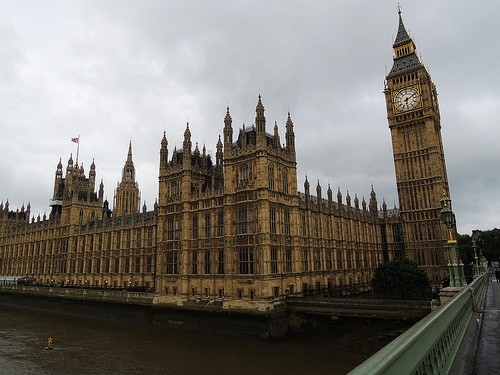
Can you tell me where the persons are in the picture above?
[495,269,500,284]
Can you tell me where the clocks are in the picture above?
[394,86,420,113]
